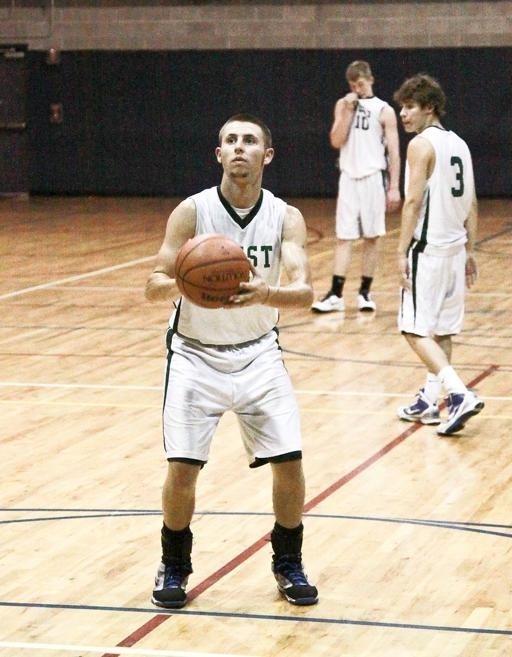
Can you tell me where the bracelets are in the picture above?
[263,281,270,307]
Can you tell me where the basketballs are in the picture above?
[175,233,250,308]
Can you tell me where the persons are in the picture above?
[144,114,320,610]
[309,61,402,315]
[394,72,485,435]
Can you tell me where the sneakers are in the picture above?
[310,292,345,313]
[397,388,440,424]
[151,562,188,607]
[357,293,376,311]
[436,391,484,434]
[271,553,319,604]
